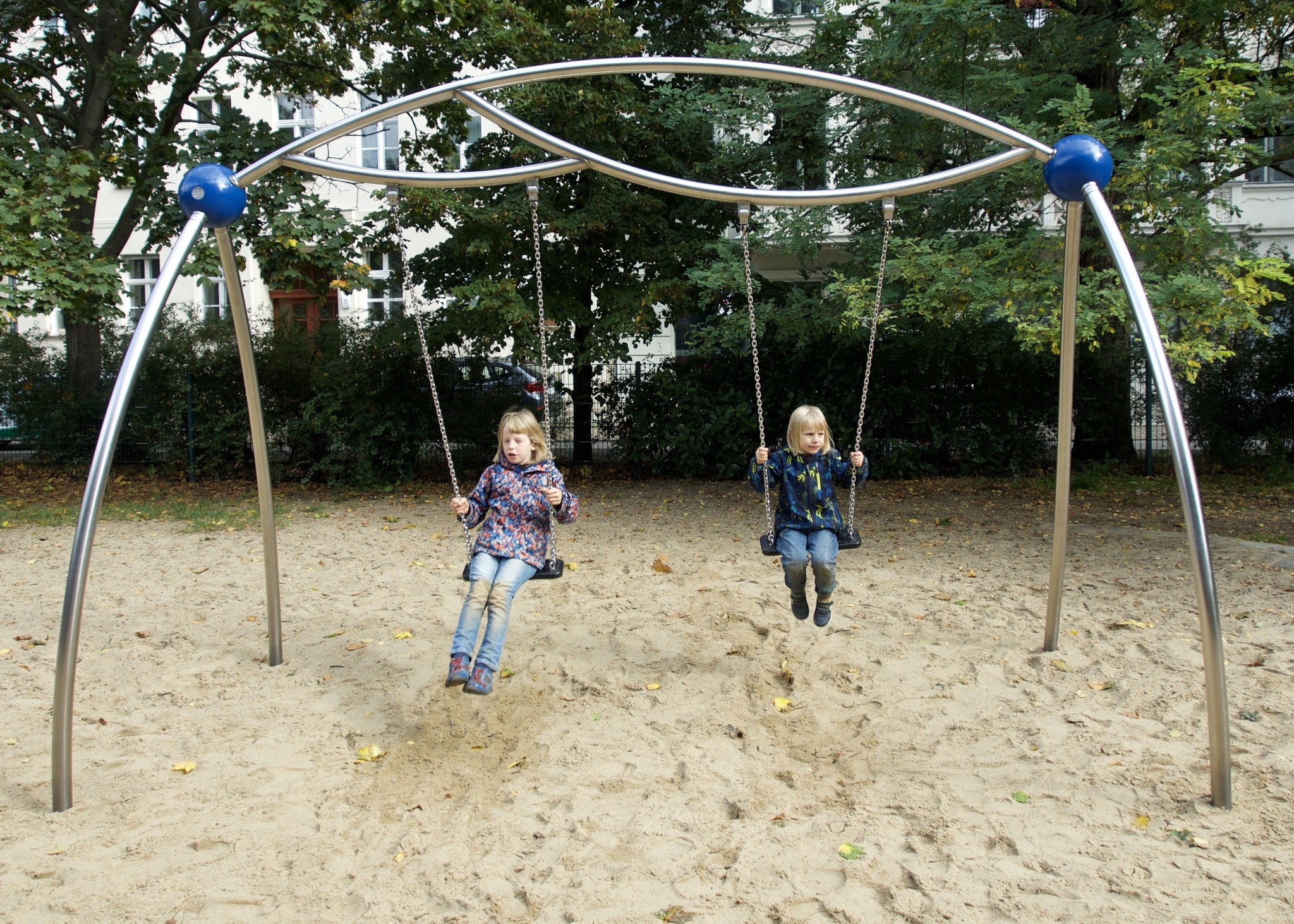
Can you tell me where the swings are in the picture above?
[735,198,898,557]
[383,184,569,582]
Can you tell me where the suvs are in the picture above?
[416,352,563,441]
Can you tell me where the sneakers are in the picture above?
[814,593,834,627]
[462,663,493,695]
[790,589,809,619]
[445,652,470,688]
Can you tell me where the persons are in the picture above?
[443,406,580,696]
[747,404,869,627]
